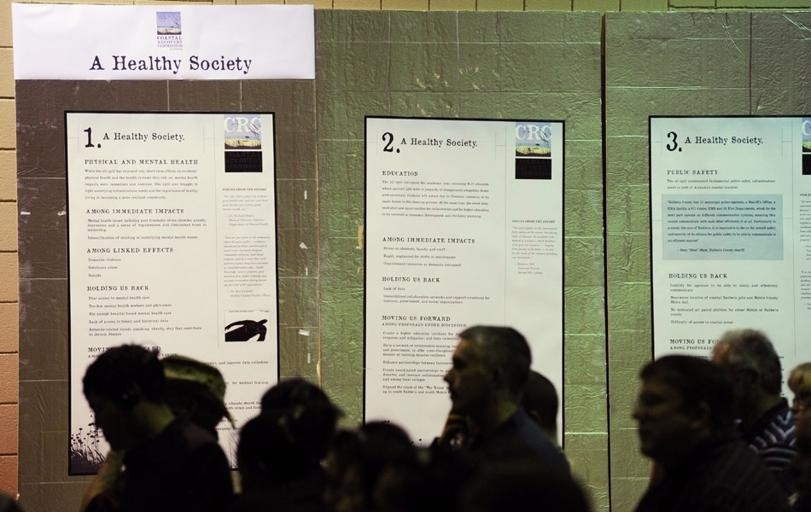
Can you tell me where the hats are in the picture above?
[260,375,346,439]
[157,354,236,438]
